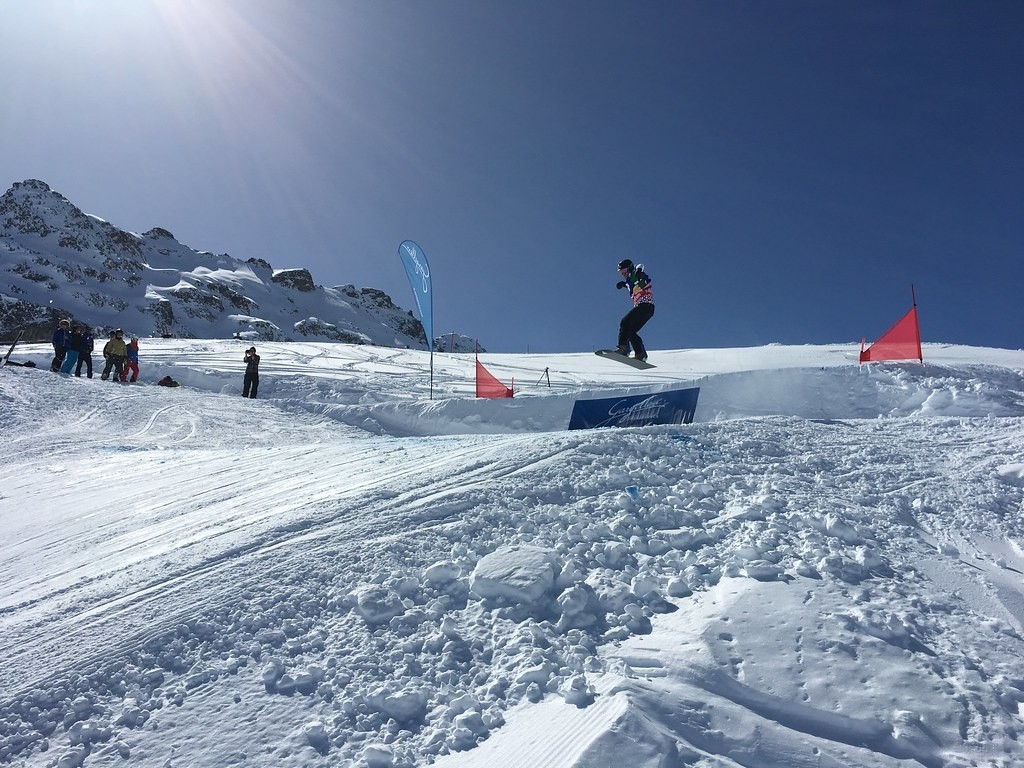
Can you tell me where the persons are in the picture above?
[50,319,94,379]
[102,328,139,382]
[234,333,242,340]
[241,347,260,399]
[615,259,655,362]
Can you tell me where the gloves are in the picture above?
[636,268,641,279]
[616,281,624,289]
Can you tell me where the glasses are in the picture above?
[619,267,628,273]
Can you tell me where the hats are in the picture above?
[617,259,634,272]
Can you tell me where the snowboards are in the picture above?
[595,348,657,370]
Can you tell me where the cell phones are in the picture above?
[246,350,250,353]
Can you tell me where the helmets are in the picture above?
[131,333,138,342]
[115,328,123,334]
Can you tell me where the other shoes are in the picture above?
[634,352,647,360]
[614,345,630,355]
